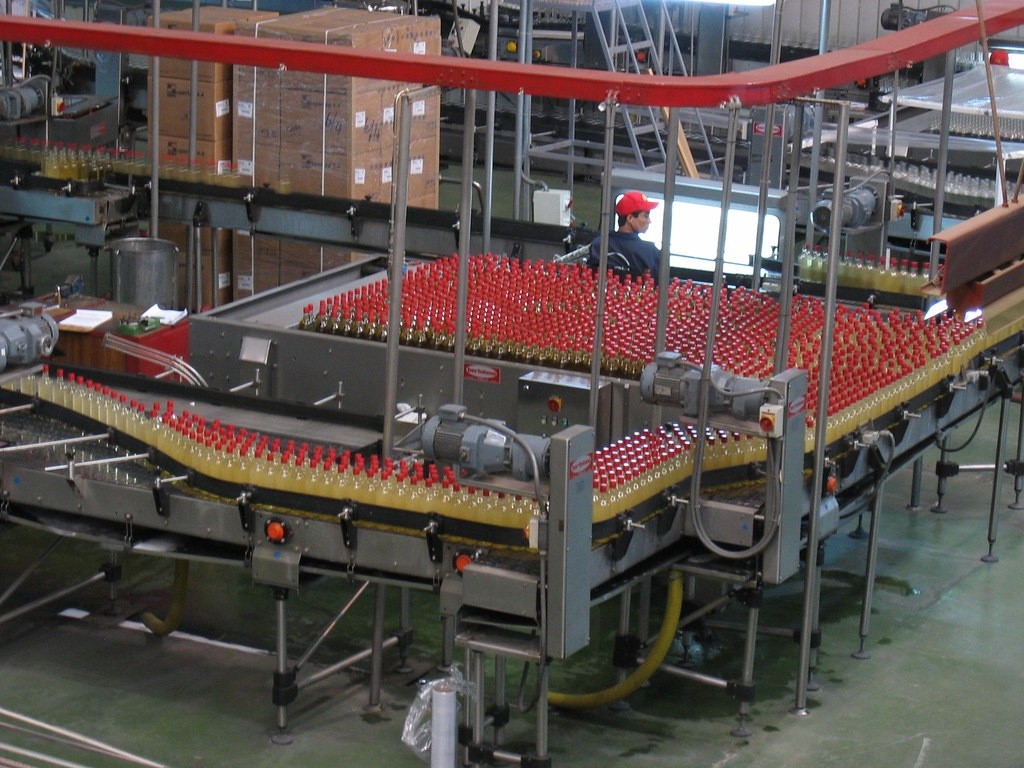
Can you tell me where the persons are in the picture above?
[587,192,660,285]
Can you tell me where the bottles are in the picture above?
[1,136,243,193]
[817,109,1024,203]
[11,241,987,540]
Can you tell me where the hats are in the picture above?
[616,192,659,214]
[989,50,1010,64]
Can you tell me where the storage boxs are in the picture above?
[145,4,445,212]
